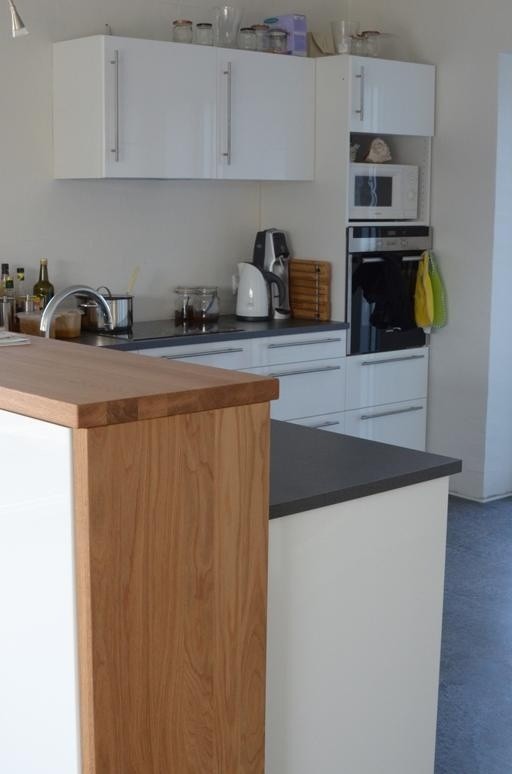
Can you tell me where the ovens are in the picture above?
[345,225,430,356]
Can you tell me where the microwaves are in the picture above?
[348,160,420,220]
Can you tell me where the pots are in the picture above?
[73,285,136,332]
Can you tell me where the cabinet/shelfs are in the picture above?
[128,329,346,453]
[316,54,436,136]
[344,345,429,452]
[215,39,316,181]
[53,33,215,179]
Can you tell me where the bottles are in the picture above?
[167,4,397,60]
[173,285,222,329]
[1,257,55,333]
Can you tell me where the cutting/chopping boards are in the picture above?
[287,259,332,323]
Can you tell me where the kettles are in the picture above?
[229,260,287,323]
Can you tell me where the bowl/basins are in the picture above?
[14,306,81,341]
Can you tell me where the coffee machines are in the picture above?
[252,225,293,322]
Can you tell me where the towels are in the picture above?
[415,251,446,334]
